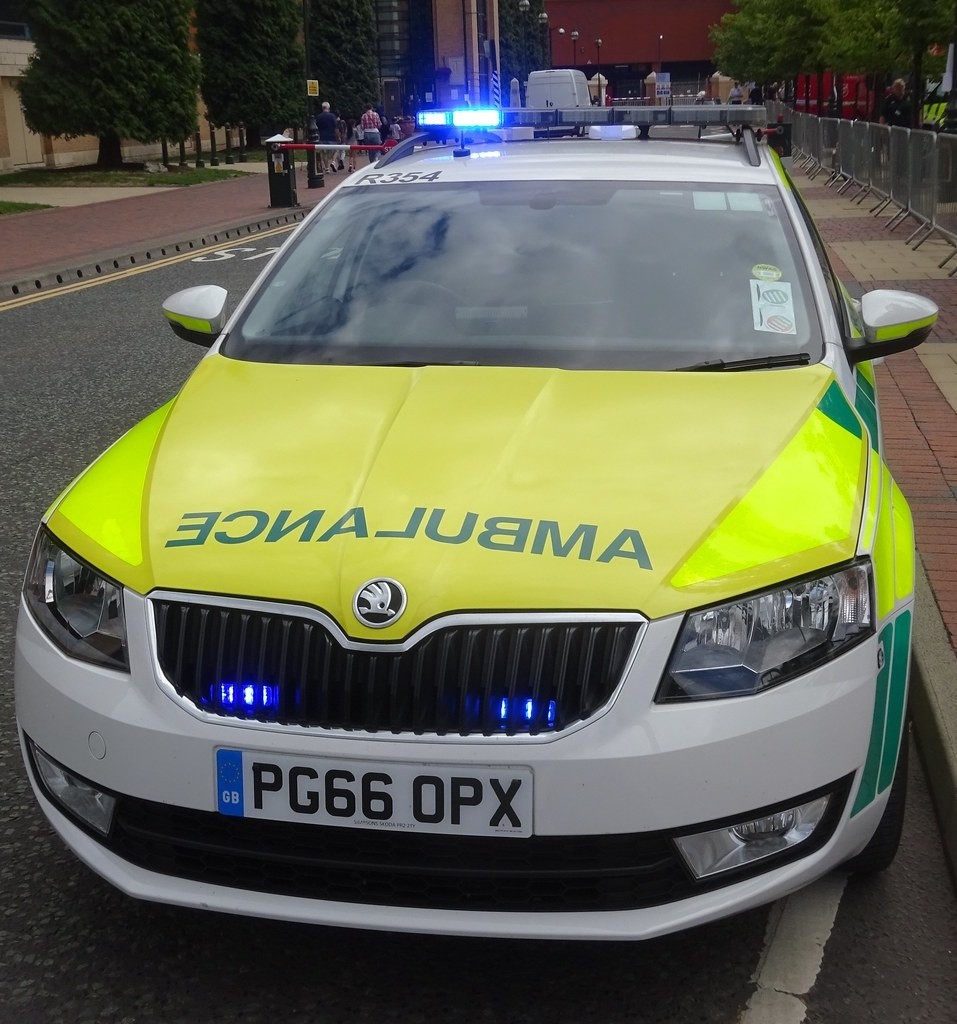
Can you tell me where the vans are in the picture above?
[525,68,601,140]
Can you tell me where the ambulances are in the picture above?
[12,102,943,940]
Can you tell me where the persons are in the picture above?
[726,81,783,105]
[879,79,913,181]
[315,100,405,173]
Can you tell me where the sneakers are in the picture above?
[325,168,331,172]
[330,161,338,172]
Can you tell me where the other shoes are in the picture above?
[338,160,344,170]
[348,164,356,172]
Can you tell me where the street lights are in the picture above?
[595,37,603,107]
[519,0,565,84]
[571,29,580,69]
